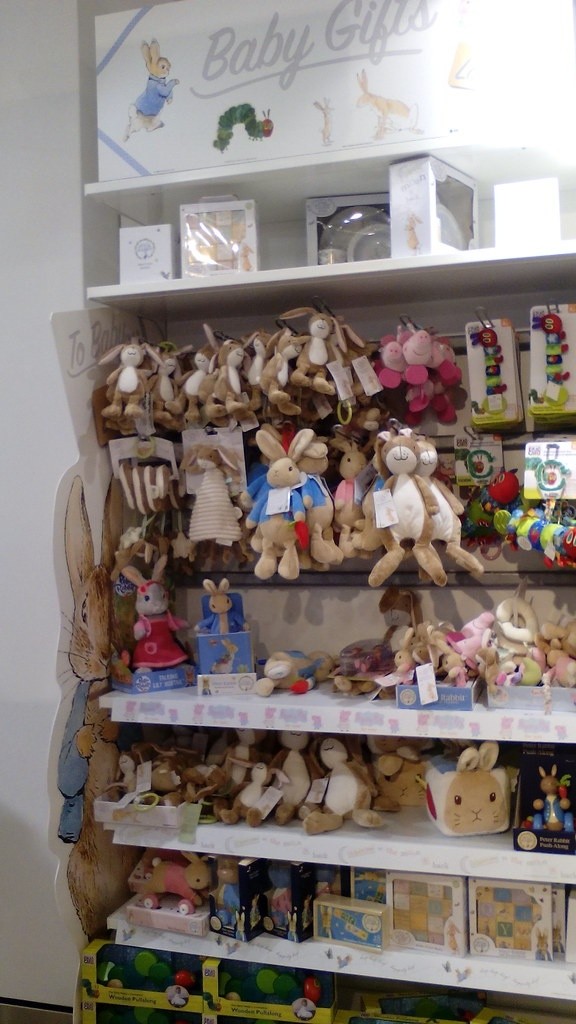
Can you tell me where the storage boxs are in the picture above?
[389,157,477,258]
[111,666,189,695]
[92,789,192,829]
[119,223,179,284]
[120,846,576,962]
[195,592,254,676]
[93,1,520,184]
[512,751,576,852]
[396,675,484,710]
[81,943,576,1024]
[486,684,576,712]
[180,193,260,277]
[305,193,390,267]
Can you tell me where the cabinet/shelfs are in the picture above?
[82,131,576,1002]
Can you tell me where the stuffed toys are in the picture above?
[122,555,191,675]
[194,578,250,634]
[350,1017,415,1024]
[382,997,473,1021]
[97,950,196,992]
[458,466,576,569]
[106,728,574,837]
[217,1015,291,1024]
[142,851,337,928]
[470,313,571,395]
[218,968,324,1005]
[96,1006,202,1024]
[98,306,484,588]
[253,583,576,696]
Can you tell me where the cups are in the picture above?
[319,249,345,265]
